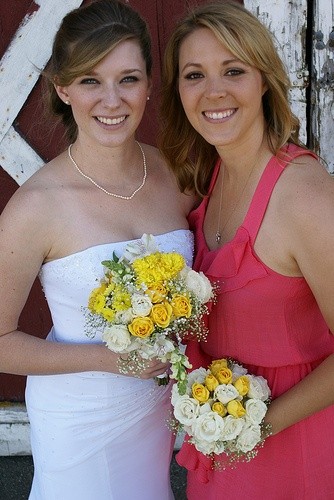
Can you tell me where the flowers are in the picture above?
[83,232,216,387]
[167,358,272,470]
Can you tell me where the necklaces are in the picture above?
[68,141,146,199]
[216,149,263,242]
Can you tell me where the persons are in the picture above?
[0,0,207,500]
[161,0,334,500]
